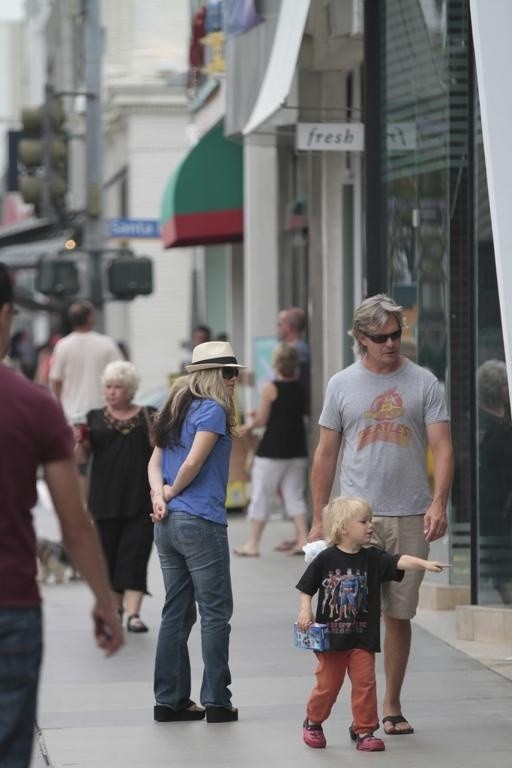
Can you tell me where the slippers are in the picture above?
[382,715,413,734]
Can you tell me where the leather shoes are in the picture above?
[127,613,148,632]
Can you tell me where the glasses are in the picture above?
[358,325,402,343]
[222,366,239,379]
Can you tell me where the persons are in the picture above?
[1,264,124,766]
[75,362,163,632]
[231,308,312,556]
[180,325,229,374]
[295,497,452,751]
[7,301,128,570]
[308,295,454,736]
[147,341,246,722]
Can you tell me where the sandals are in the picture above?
[303,717,326,748]
[349,726,386,752]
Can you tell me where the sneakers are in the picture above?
[207,705,238,723]
[154,701,205,722]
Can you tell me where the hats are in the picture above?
[185,341,248,374]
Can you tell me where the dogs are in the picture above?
[34,538,78,584]
